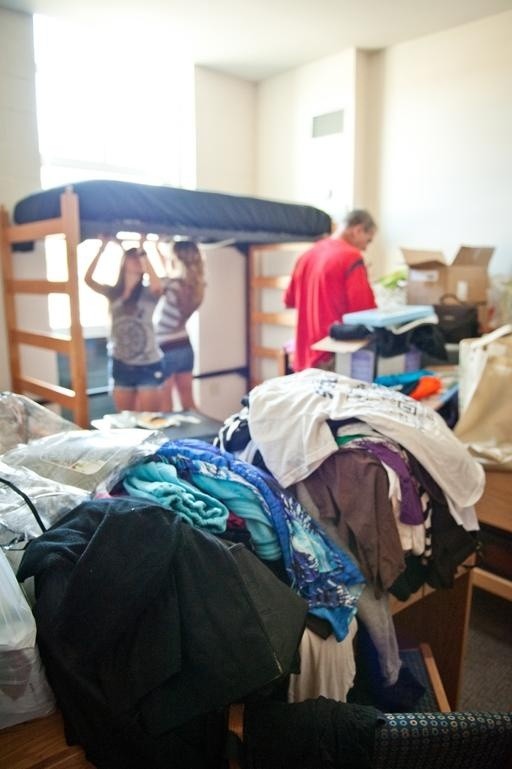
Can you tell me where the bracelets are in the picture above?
[138,252,146,258]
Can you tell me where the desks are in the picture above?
[469,466,512,612]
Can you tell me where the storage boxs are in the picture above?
[306,336,424,385]
[342,302,436,331]
[400,245,496,330]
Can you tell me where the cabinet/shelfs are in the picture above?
[0,546,482,769]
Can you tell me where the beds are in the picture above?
[1,179,336,432]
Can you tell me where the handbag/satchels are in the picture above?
[434,293,479,343]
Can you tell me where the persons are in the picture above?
[284,211,379,374]
[112,236,206,414]
[85,235,171,413]
